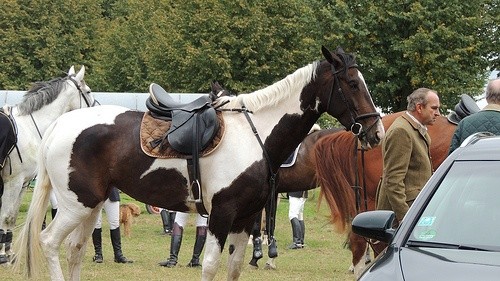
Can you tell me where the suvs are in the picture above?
[351,129,500,281]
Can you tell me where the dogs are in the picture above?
[118,203,139,239]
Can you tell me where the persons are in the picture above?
[447,79,500,156]
[92,186,133,263]
[375,88,440,230]
[42,190,58,230]
[159,211,209,268]
[159,207,176,235]
[288,190,308,250]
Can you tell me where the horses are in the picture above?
[11,42,379,280]
[243,106,456,275]
[0,61,103,280]
